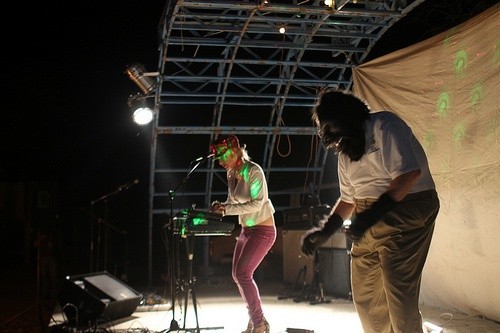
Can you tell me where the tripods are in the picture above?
[0,231,55,329]
[158,158,207,333]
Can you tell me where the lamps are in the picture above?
[124,62,160,127]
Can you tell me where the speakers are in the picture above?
[282,230,353,302]
[56,270,144,326]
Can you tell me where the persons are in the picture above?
[300,90,440,333]
[210,135,277,333]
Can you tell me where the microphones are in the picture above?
[116,179,139,189]
[194,152,215,162]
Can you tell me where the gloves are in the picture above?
[300,214,343,259]
[348,192,396,240]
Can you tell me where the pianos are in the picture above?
[168,207,236,333]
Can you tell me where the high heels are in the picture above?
[241,316,270,333]
